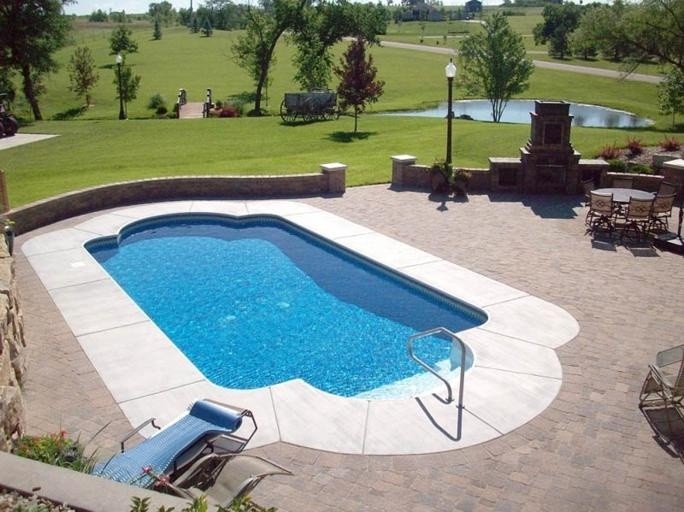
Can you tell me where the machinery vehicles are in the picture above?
[0,90,18,137]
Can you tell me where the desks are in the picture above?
[591,185,657,232]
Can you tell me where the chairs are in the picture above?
[584,188,619,242]
[652,192,676,236]
[652,180,679,232]
[617,196,654,245]
[145,448,292,512]
[612,175,636,229]
[90,399,257,491]
[579,177,603,228]
[638,345,684,443]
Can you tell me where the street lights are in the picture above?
[114,53,128,119]
[444,57,458,167]
